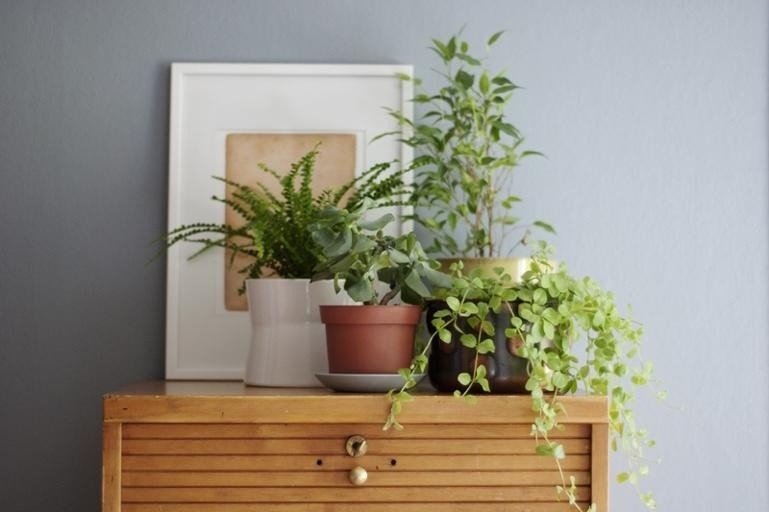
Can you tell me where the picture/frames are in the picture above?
[164,62,417,382]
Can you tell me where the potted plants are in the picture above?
[157,139,431,388]
[306,25,660,512]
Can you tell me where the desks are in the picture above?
[101,379,611,511]
